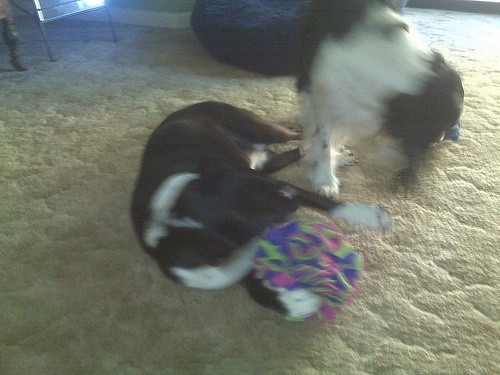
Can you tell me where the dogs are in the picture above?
[295,0,465,201]
[129,101,394,319]
